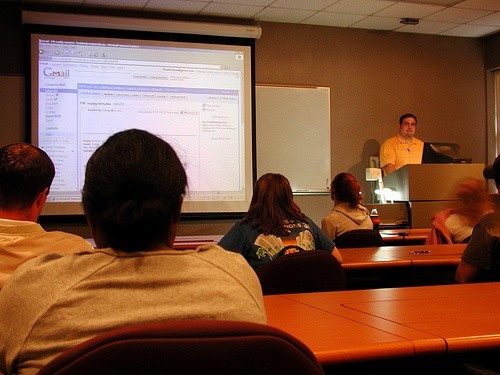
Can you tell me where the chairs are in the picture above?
[256,251,346,296]
[34,320,324,375]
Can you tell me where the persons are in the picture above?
[217,172,342,285]
[455,154,500,284]
[426,176,500,245]
[379,113,425,178]
[321,172,373,242]
[1,142,94,292]
[0,128,268,375]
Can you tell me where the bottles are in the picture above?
[370,208,380,233]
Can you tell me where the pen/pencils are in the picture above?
[410,250,430,254]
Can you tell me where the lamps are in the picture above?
[366,168,387,204]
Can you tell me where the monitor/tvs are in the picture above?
[422,142,472,164]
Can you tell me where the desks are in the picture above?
[86,228,432,250]
[264,281,500,365]
[337,243,468,269]
